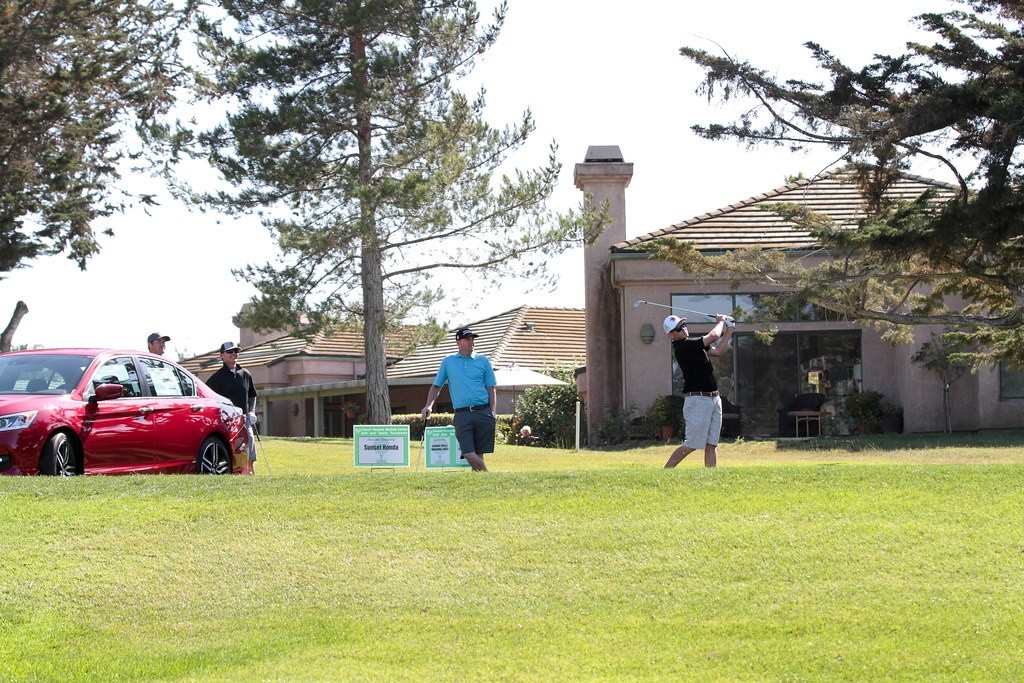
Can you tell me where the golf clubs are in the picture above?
[634,299,736,325]
[415,411,428,471]
[253,423,272,476]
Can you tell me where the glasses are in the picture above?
[669,322,686,332]
[226,348,238,353]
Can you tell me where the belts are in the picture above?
[686,391,719,396]
[453,404,489,412]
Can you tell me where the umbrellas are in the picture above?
[491,360,570,416]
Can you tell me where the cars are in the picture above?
[0,349,253,477]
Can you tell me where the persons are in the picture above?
[663,313,735,468]
[147,333,170,357]
[205,342,257,474]
[422,328,497,472]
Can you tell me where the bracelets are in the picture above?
[490,408,496,412]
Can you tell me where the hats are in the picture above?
[663,315,687,334]
[148,333,170,347]
[456,329,479,340]
[220,342,242,354]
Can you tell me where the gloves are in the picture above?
[724,316,736,329]
[247,411,258,425]
